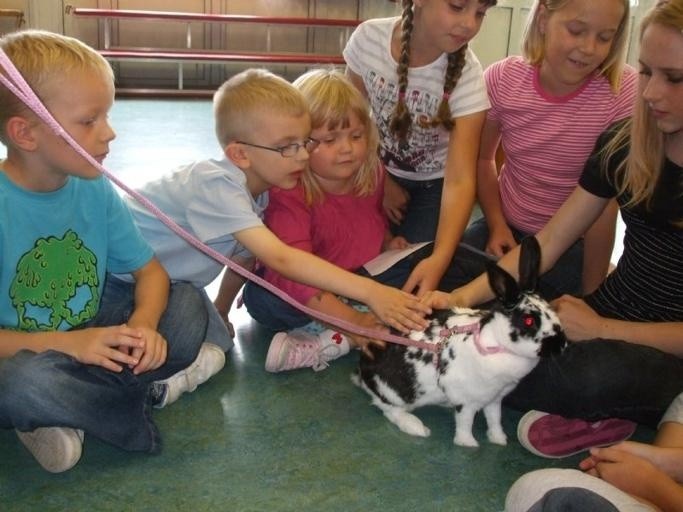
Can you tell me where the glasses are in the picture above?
[236,136,321,158]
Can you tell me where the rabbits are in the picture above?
[347,232,569,450]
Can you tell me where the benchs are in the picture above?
[70,7,361,100]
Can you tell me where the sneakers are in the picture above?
[265,326,349,372]
[518,408,637,459]
[151,342,226,408]
[504,467,658,512]
[14,426,85,474]
